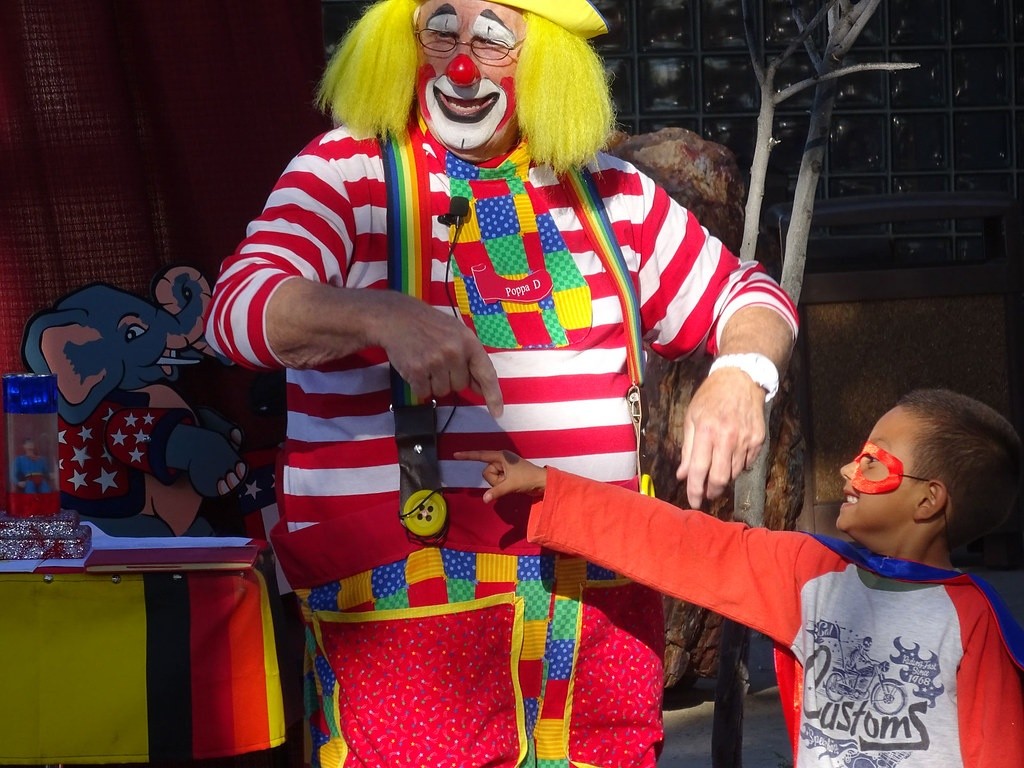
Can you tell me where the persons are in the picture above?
[453,389,1024,768]
[12,436,57,493]
[203,0,799,768]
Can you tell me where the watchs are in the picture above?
[708,353,780,403]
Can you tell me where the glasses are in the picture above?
[411,21,525,60]
